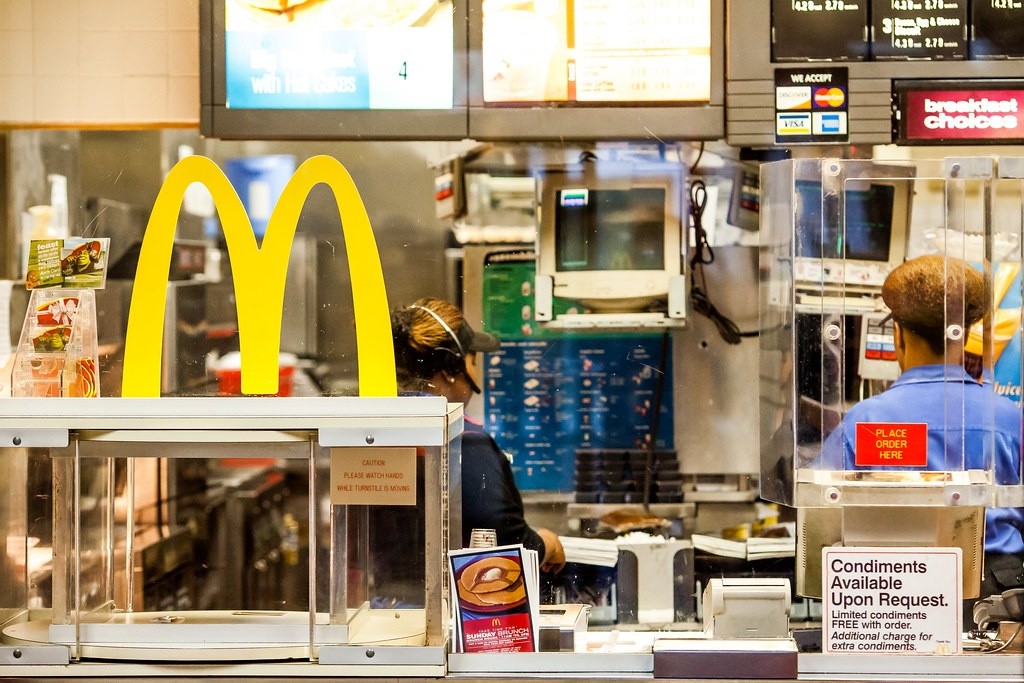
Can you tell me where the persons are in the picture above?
[807,256,1024,634]
[370,297,568,606]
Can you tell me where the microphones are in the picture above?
[461,368,482,393]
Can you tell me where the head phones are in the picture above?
[404,305,466,376]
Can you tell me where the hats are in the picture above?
[393,316,500,373]
[877,254,991,330]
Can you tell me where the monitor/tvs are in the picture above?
[468,0,726,143]
[198,0,468,142]
[793,157,913,294]
[539,171,681,312]
